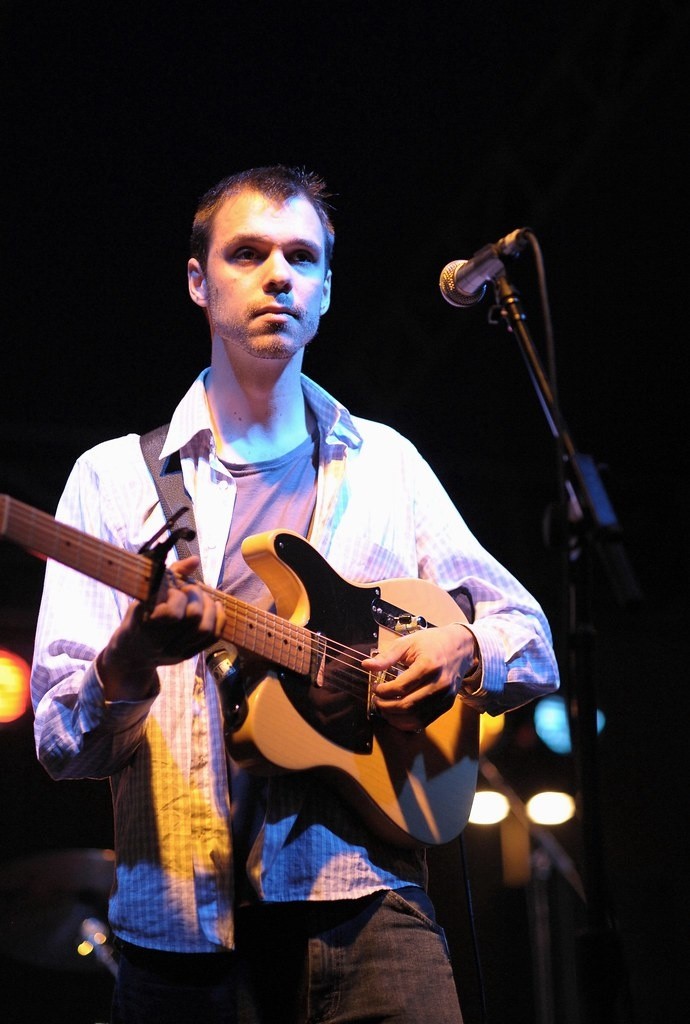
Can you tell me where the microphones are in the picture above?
[439,227,530,308]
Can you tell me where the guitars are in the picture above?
[0,492,481,854]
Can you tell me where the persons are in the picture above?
[31,164,562,1024]
